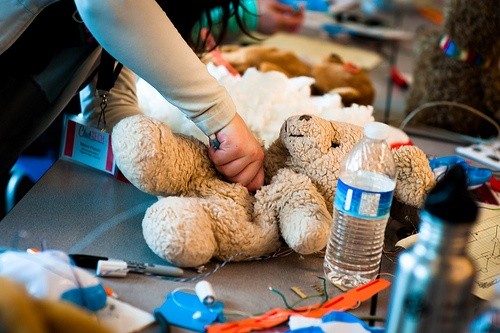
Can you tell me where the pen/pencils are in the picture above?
[27,248,118,298]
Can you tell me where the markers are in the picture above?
[392,72,408,90]
[68,248,183,276]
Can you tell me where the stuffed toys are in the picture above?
[111,113,436,268]
[406,0,500,139]
[200,44,375,107]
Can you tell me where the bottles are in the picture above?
[323,120,399,289]
[384,164,480,333]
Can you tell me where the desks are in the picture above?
[0,19,500,333]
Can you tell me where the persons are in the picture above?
[192,0,306,51]
[0,0,265,221]
